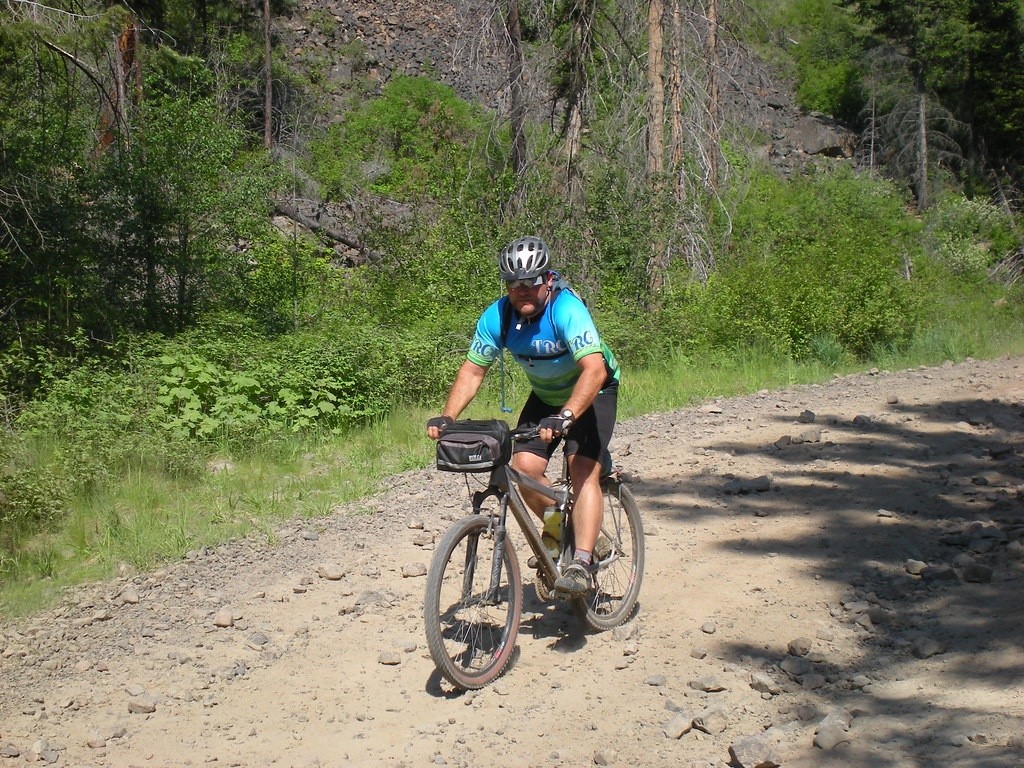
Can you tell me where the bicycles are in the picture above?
[423,418,645,691]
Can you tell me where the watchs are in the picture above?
[558,408,576,421]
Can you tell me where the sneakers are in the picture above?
[528,556,540,568]
[554,559,595,596]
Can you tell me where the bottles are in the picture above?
[542,504,563,557]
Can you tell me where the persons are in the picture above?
[426,234,622,595]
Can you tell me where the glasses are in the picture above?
[506,275,543,291]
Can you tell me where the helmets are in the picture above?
[499,236,551,280]
